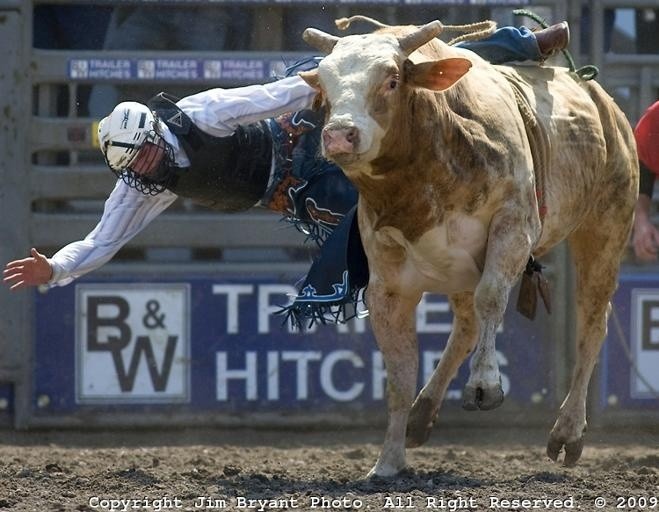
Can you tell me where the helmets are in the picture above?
[96,99,176,197]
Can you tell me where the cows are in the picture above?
[297,17,645,484]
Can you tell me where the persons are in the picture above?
[2,19,571,296]
[630,99,657,267]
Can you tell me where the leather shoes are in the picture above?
[533,20,570,56]
